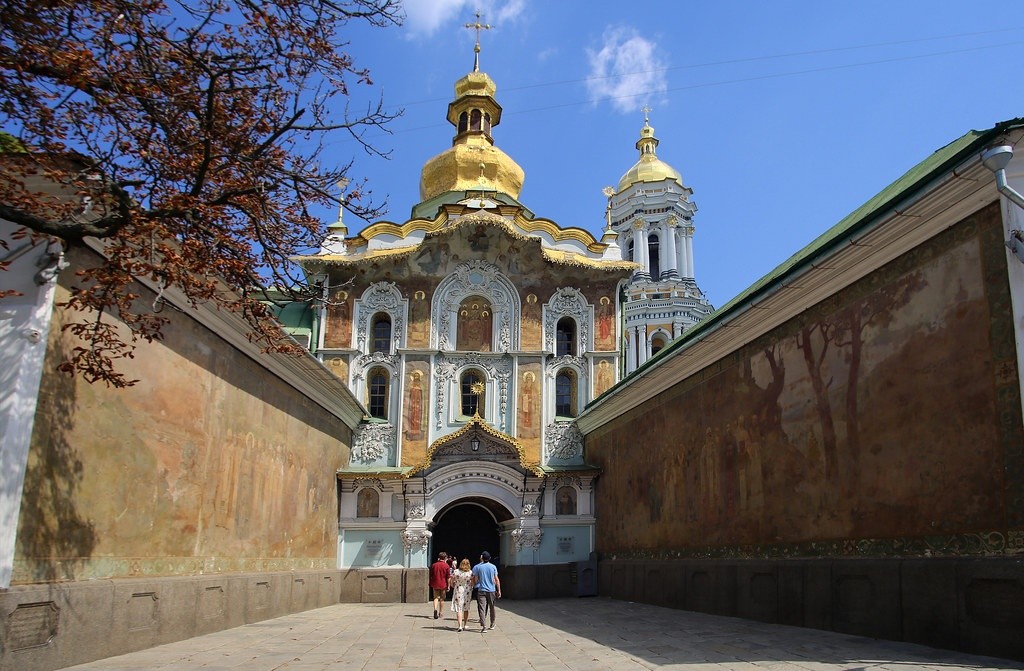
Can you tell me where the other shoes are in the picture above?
[463,626,469,631]
[490,623,496,629]
[434,610,438,619]
[458,628,462,632]
[481,627,487,632]
[439,613,444,618]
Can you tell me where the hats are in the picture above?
[481,551,490,561]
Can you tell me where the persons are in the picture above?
[429,552,450,619]
[451,559,473,632]
[446,556,488,626]
[472,551,501,633]
